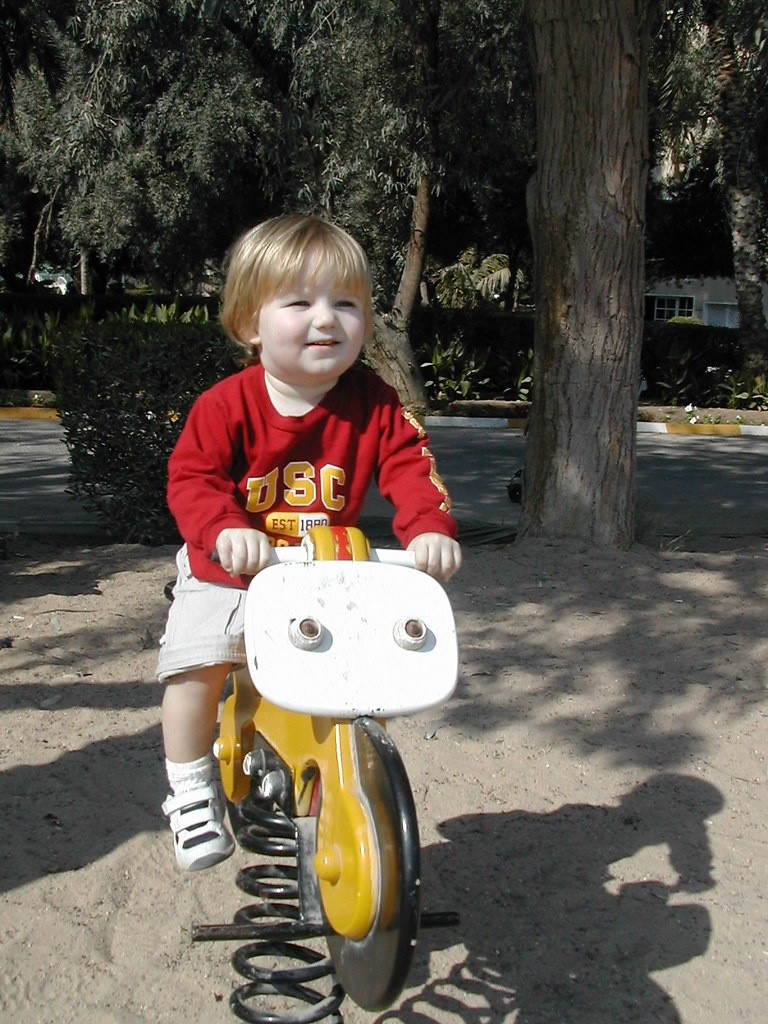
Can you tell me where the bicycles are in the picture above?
[158,526,460,1011]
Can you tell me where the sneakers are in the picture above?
[162,780,235,871]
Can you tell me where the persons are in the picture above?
[158,214,461,871]
